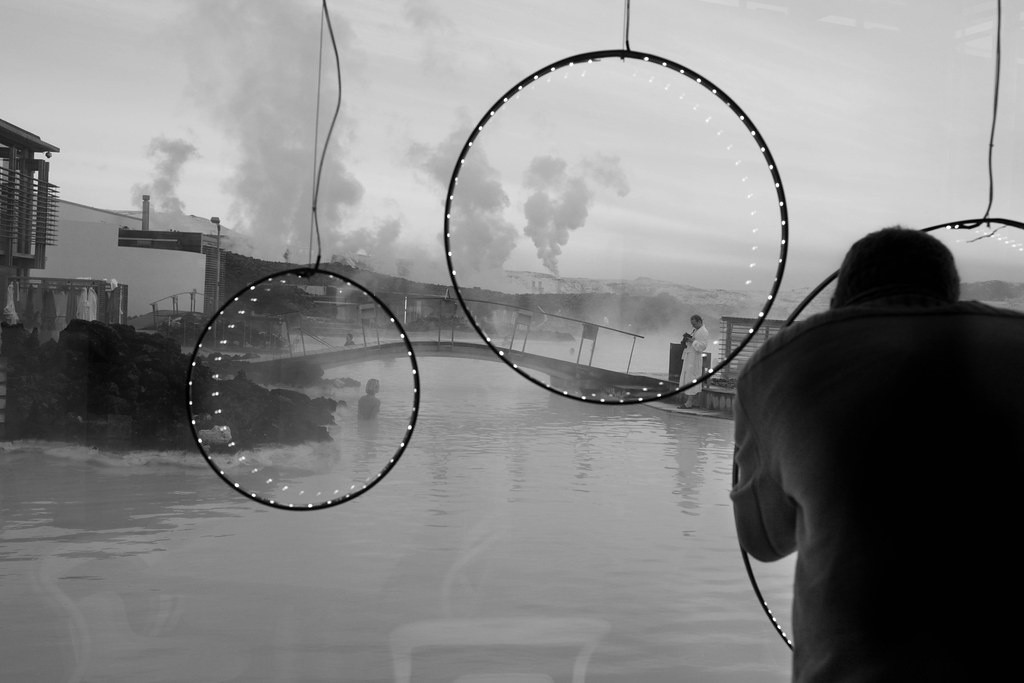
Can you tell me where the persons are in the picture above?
[729,222,1021,683]
[354,378,382,420]
[676,312,709,407]
[343,332,357,350]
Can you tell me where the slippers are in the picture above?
[692,405,700,408]
[677,403,692,409]
